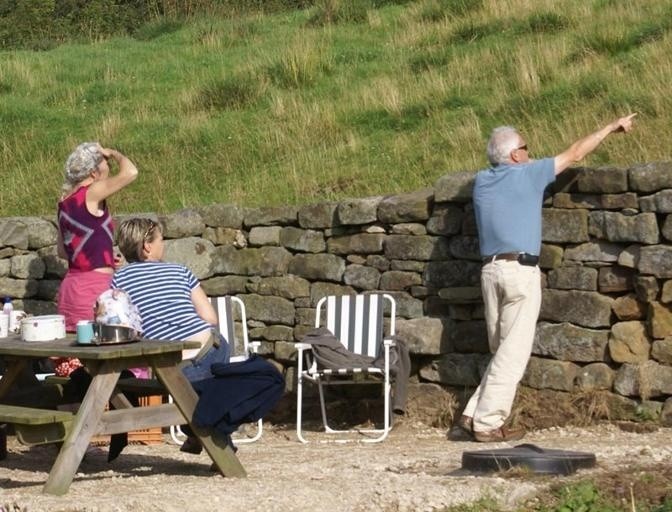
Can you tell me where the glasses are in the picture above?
[143,219,157,249]
[519,144,527,150]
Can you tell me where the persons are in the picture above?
[54,141,140,333]
[453,112,638,442]
[104,217,239,473]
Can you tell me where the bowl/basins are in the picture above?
[101,324,135,344]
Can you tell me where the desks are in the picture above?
[0,333,248,494]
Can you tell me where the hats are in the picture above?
[95,289,143,333]
[65,143,105,183]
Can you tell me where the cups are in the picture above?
[0,309,27,338]
[76,319,99,344]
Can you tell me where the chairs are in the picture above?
[166,294,265,447]
[293,292,398,445]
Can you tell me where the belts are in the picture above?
[482,253,519,266]
[179,330,220,368]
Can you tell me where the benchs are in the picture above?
[0,404,75,494]
[46,376,248,479]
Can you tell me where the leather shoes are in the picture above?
[474,427,525,442]
[458,415,473,436]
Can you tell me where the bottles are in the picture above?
[3,298,14,328]
